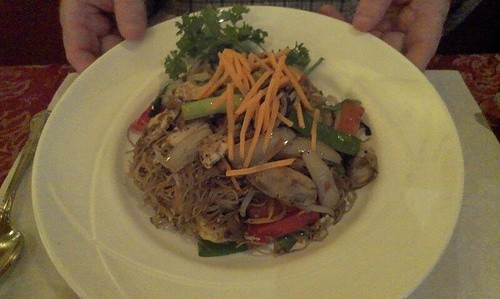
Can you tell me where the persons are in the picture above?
[59,0,450,75]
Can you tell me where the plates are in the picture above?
[31,7,463,299]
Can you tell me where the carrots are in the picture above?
[195,50,321,176]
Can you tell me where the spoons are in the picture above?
[0,110,51,287]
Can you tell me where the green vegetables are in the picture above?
[162,4,310,78]
